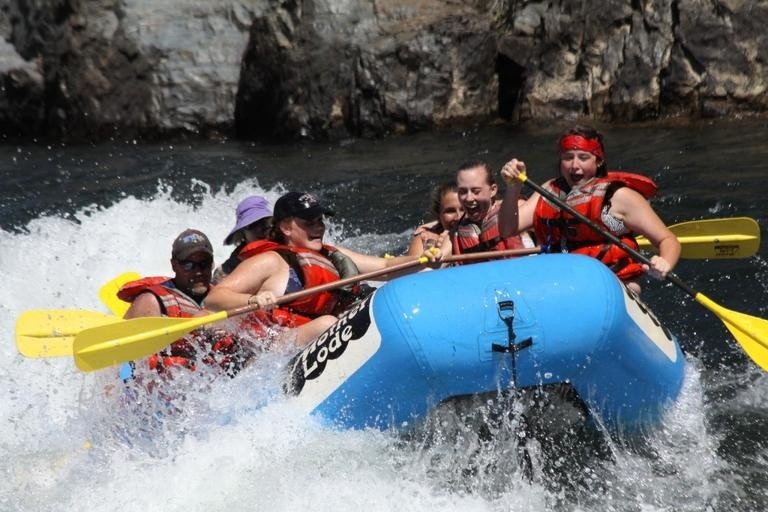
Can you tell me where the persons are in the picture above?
[202,193,444,370]
[212,195,275,286]
[123,228,240,414]
[421,159,537,272]
[498,125,682,294]
[407,180,465,256]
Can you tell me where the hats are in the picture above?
[273,190,333,221]
[224,195,274,243]
[172,229,213,261]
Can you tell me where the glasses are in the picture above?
[181,257,213,271]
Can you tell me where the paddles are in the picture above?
[73,248,443,372]
[15,309,241,357]
[100,271,145,316]
[384,218,761,258]
[503,161,767,374]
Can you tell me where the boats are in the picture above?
[116,247,684,482]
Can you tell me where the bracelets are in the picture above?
[248,294,254,305]
